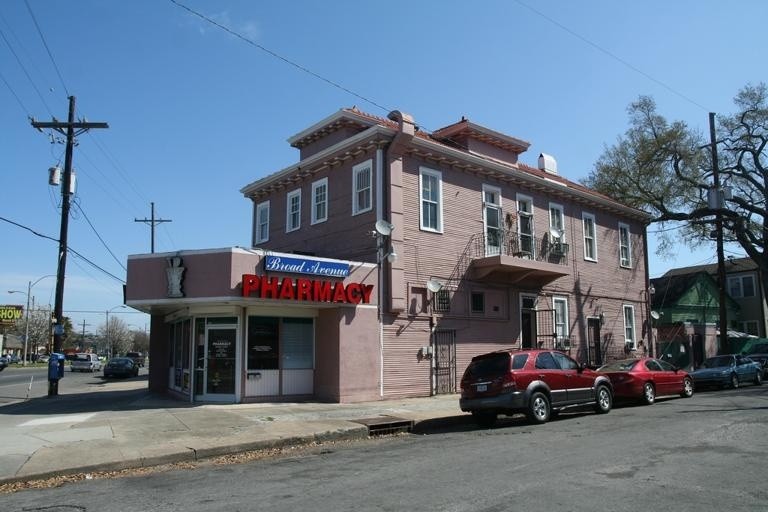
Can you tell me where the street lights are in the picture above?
[646,283,655,357]
[7,274,56,367]
[104,304,127,361]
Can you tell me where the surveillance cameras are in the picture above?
[650,288,655,294]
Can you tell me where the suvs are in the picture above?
[456,345,614,427]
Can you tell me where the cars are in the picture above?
[593,353,768,404]
[0,349,145,378]
